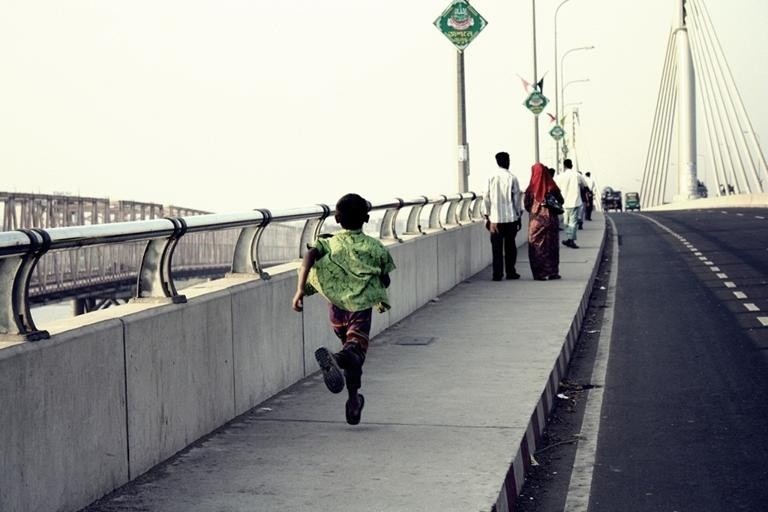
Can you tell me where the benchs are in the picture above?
[75,284,138,320]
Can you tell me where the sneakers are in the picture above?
[534,275,562,280]
[561,238,579,249]
[345,392,365,426]
[314,345,345,395]
[492,273,521,281]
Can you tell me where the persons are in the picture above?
[481,151,523,281]
[549,158,595,248]
[524,162,563,280]
[293,194,396,423]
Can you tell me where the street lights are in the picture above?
[558,45,595,177]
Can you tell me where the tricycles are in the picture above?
[624,192,640,213]
[600,189,622,213]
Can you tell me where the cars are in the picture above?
[698,184,708,198]
[718,184,735,196]
